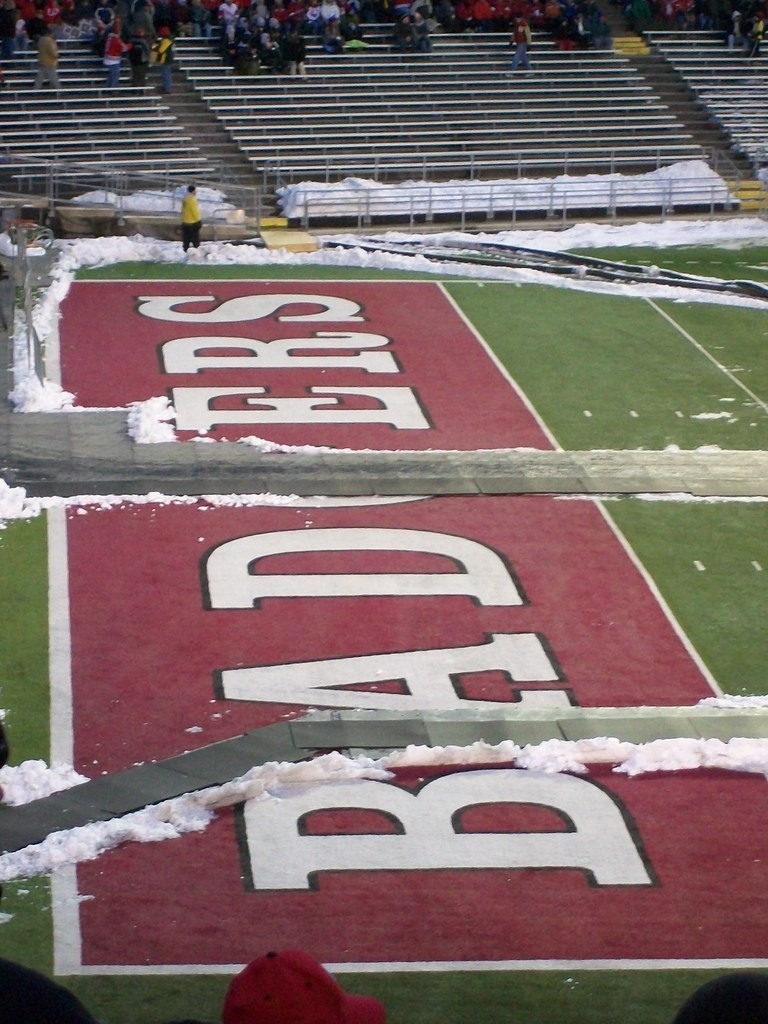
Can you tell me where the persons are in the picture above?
[619,0,768,64]
[33,27,64,95]
[103,22,133,93]
[1,0,364,81]
[502,10,535,78]
[180,185,204,251]
[384,1,613,57]
[220,947,385,1024]
[126,27,152,96]
[149,27,177,93]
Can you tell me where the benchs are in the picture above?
[1,26,767,182]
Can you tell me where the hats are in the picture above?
[221,949,389,1024]
